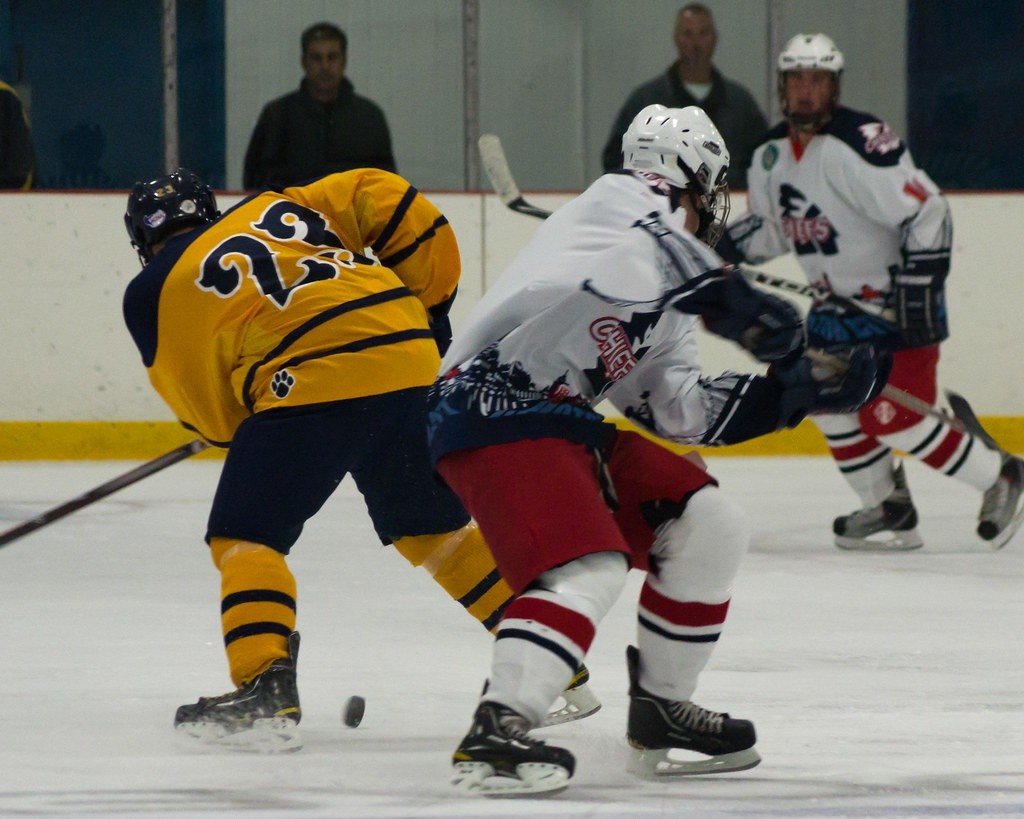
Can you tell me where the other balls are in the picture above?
[344,696,366,728]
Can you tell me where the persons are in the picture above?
[242,21,398,193]
[601,5,771,192]
[122,167,601,752]
[418,103,893,799]
[0,80,39,192]
[703,33,1024,551]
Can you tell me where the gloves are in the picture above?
[728,294,807,362]
[886,279,942,340]
[799,341,893,418]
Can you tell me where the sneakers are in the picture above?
[450,677,577,792]
[173,631,301,753]
[832,459,923,551]
[976,460,1024,552]
[624,644,761,774]
[532,664,601,727]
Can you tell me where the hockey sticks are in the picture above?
[803,345,996,445]
[475,130,899,321]
[0,435,212,545]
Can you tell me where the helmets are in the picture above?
[124,166,220,267]
[775,32,846,74]
[621,105,730,249]
